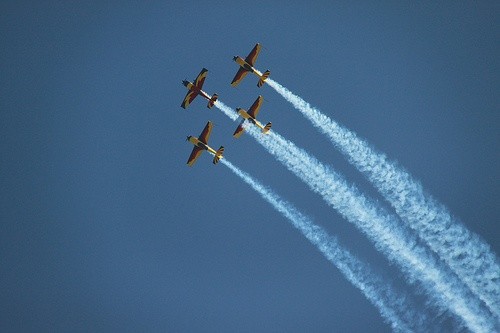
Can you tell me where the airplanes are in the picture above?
[231,94,272,140]
[183,119,224,169]
[229,40,271,91]
[180,67,219,110]
